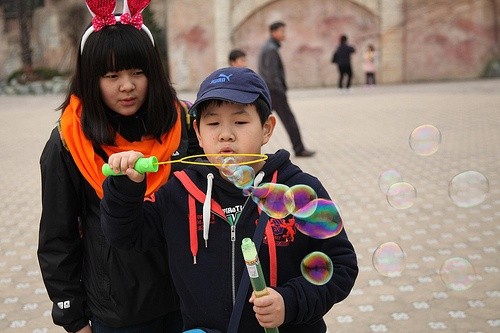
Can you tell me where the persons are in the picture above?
[254,21,315,157]
[36,12,202,333]
[100,66,359,333]
[331,34,355,88]
[362,44,377,84]
[228,50,247,67]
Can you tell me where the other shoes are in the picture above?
[296,149,315,157]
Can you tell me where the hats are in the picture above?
[187,66,273,118]
